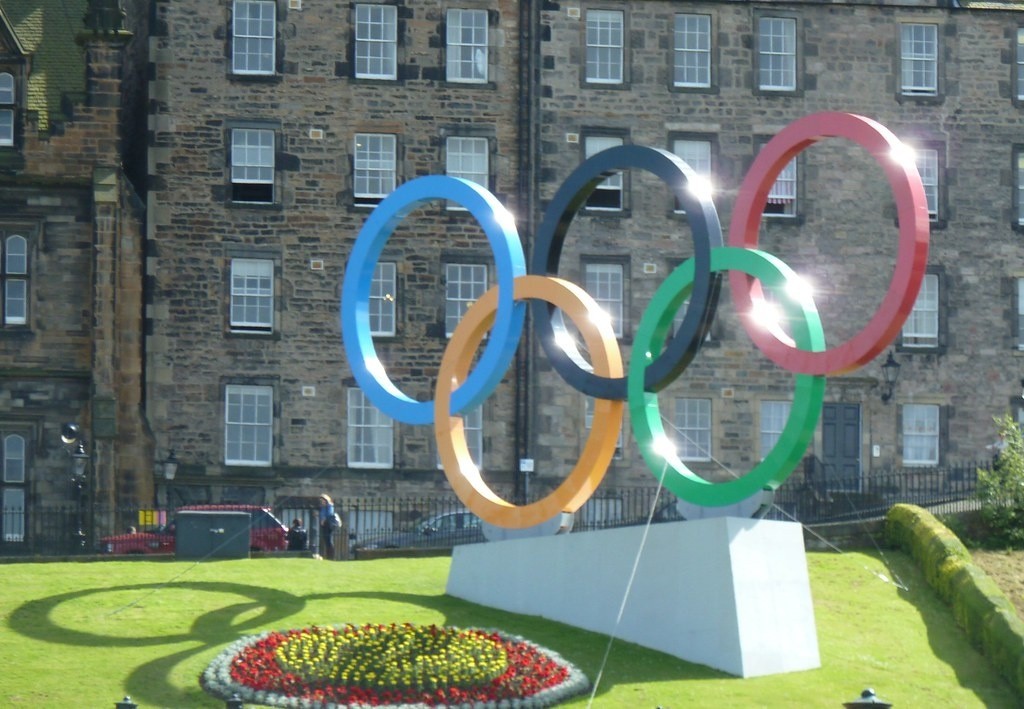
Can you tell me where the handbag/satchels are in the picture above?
[325,503,342,530]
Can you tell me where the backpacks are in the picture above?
[293,526,306,548]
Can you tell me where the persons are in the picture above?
[281,518,309,551]
[126,526,136,534]
[432,518,456,532]
[320,493,335,560]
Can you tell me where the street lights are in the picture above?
[71,439,90,557]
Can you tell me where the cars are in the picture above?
[347,507,485,558]
[95,505,293,555]
